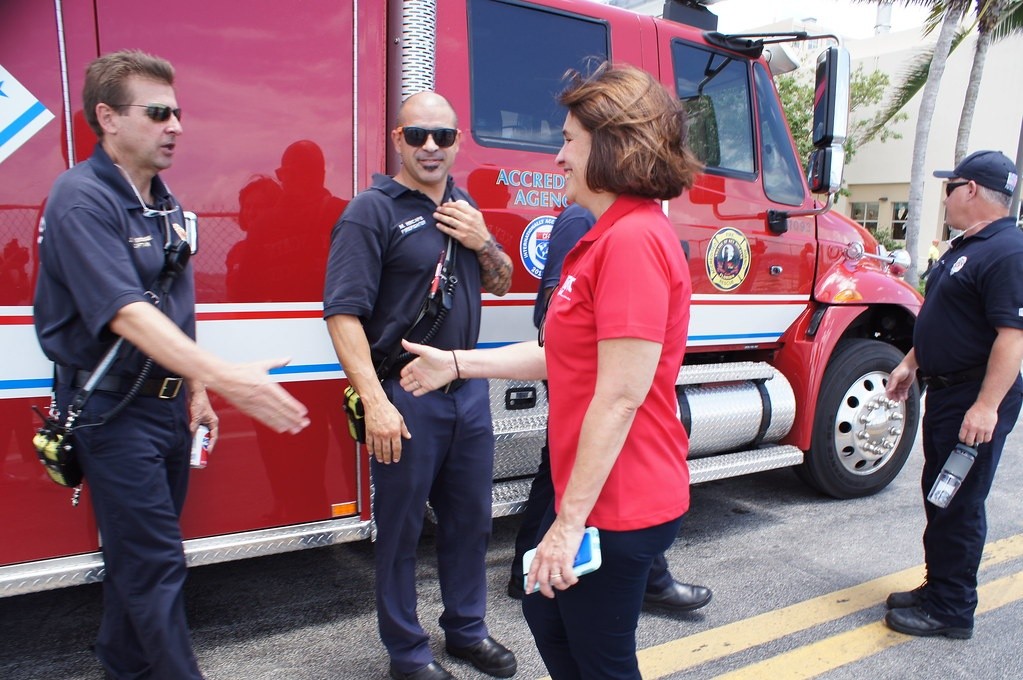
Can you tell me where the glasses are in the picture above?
[397,126,461,148]
[113,164,179,217]
[109,104,181,122]
[946,182,968,196]
[538,283,559,346]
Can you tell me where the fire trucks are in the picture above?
[0,2,933,600]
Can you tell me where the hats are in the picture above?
[933,150,1018,195]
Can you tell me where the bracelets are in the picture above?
[452,350,459,379]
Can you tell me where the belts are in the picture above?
[56,365,183,399]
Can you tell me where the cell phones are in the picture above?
[523,526,601,594]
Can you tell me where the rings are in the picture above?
[551,574,561,578]
[415,381,420,385]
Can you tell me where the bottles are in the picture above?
[927,440,982,508]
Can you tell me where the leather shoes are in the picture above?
[886,606,972,639]
[643,580,712,610]
[508,573,525,599]
[887,580,927,608]
[446,636,517,677]
[390,660,457,680]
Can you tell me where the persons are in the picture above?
[509,206,713,610]
[35,50,311,679]
[323,91,517,679]
[886,150,1022,639]
[919,240,939,282]
[401,67,703,680]
